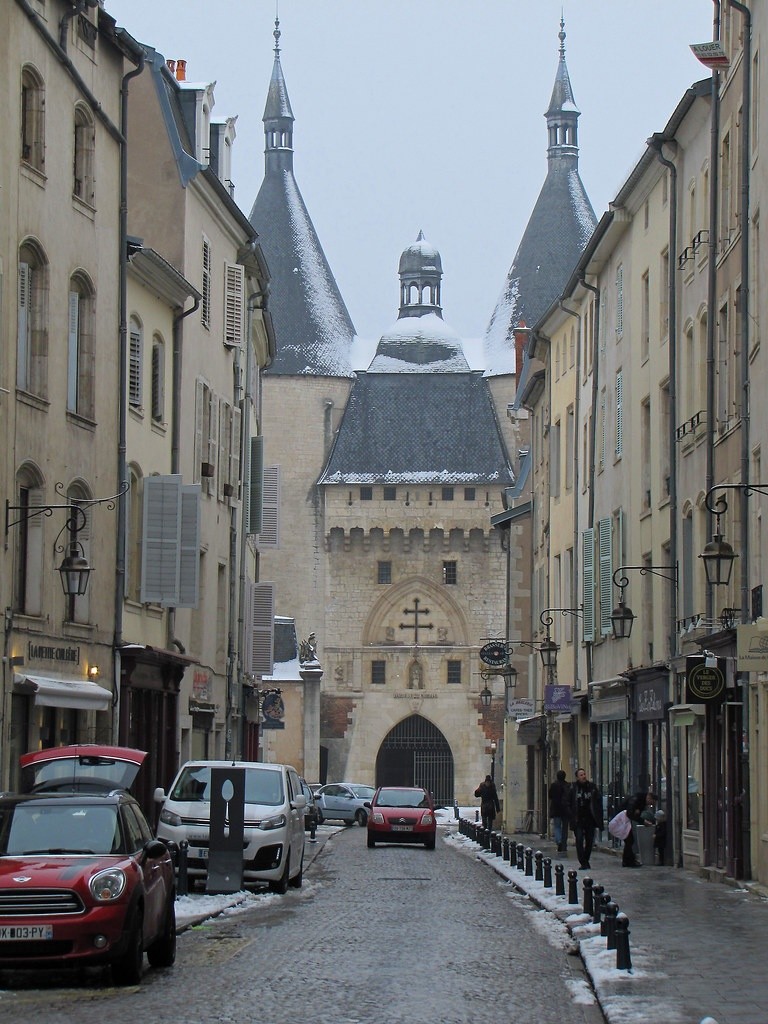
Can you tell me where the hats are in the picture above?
[486,776,491,782]
[654,810,667,822]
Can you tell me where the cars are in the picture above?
[312,783,377,827]
[299,778,323,832]
[363,785,442,850]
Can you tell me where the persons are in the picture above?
[474,775,500,838]
[652,810,667,866]
[562,768,604,870]
[607,771,624,847]
[616,791,659,867]
[548,770,571,851]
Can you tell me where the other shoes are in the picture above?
[658,863,664,866]
[585,862,590,868]
[578,864,586,870]
[623,860,641,867]
[558,841,562,852]
[563,847,567,851]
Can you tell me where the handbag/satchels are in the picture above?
[608,810,631,840]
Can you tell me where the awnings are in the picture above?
[14,671,113,711]
[515,714,546,733]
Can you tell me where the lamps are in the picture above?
[478,668,507,706]
[501,640,544,688]
[4,499,96,597]
[540,604,584,668]
[608,560,679,639]
[698,483,768,588]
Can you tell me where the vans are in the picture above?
[152,759,308,894]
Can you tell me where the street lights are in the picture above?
[490,739,497,783]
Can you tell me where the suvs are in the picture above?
[0,744,177,988]
